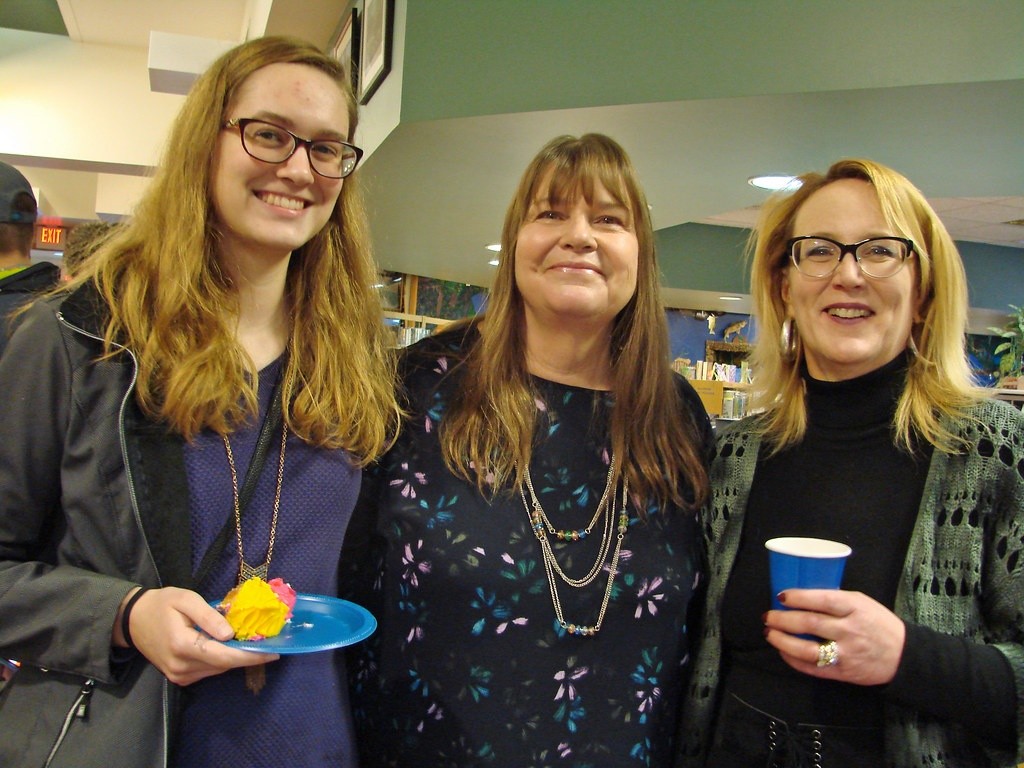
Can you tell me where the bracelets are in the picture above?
[120,587,154,652]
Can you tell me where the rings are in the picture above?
[816,640,838,668]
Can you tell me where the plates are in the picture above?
[198,596,378,657]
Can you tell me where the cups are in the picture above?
[764,534,850,643]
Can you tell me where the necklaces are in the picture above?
[510,441,632,638]
[219,409,286,700]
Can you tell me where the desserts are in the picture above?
[215,576,296,641]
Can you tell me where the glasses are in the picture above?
[219,118,364,180]
[785,235,915,280]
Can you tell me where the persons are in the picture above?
[0,161,61,357]
[672,157,1023,768]
[337,129,717,768]
[0,32,415,768]
[60,223,125,282]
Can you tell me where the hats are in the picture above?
[0,161,38,224]
